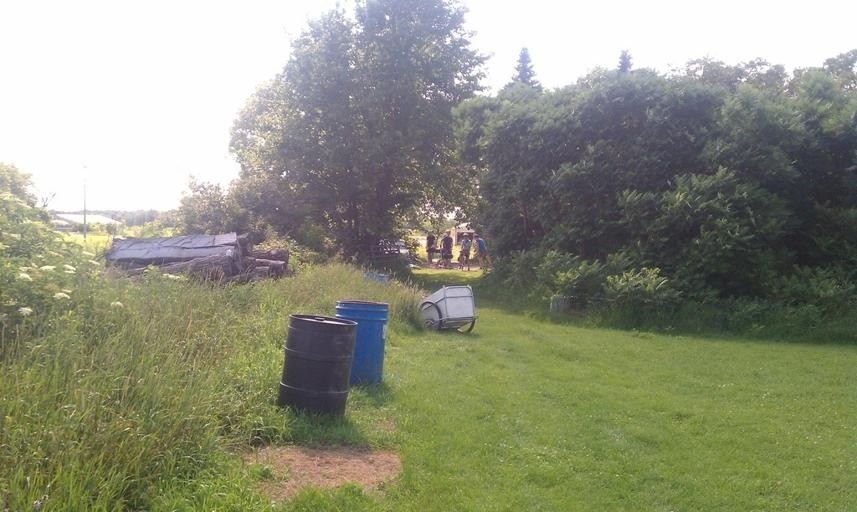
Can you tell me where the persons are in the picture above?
[458,233,472,271]
[473,233,488,269]
[426,230,436,262]
[439,230,453,265]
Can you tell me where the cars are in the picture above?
[378,238,409,258]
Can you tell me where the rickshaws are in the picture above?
[416,283,479,335]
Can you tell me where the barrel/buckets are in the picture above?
[275,311,359,419]
[336,300,390,389]
